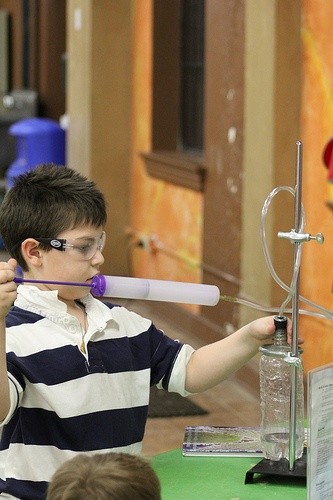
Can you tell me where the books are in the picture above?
[182,426,303,458]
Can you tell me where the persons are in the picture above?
[0,161,293,500]
[46,452,161,500]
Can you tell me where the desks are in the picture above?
[151,418,308,500]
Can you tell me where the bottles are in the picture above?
[259,338,305,461]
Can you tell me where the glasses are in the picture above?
[10,231,106,260]
